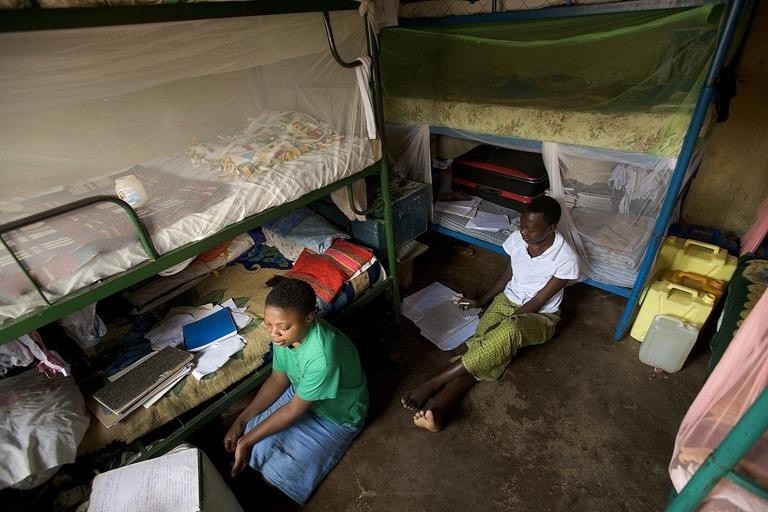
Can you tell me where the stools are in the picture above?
[84,447,242,512]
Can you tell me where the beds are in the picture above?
[1,0,408,512]
[663,199,768,512]
[380,2,744,344]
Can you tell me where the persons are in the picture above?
[224,279,369,512]
[401,196,578,432]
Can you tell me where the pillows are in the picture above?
[186,106,342,184]
[261,227,304,266]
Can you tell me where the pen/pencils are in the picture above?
[450,301,471,305]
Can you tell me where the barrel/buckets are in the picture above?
[630,265,716,343]
[638,314,700,376]
[668,219,741,256]
[638,236,738,308]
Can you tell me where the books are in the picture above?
[182,307,238,352]
